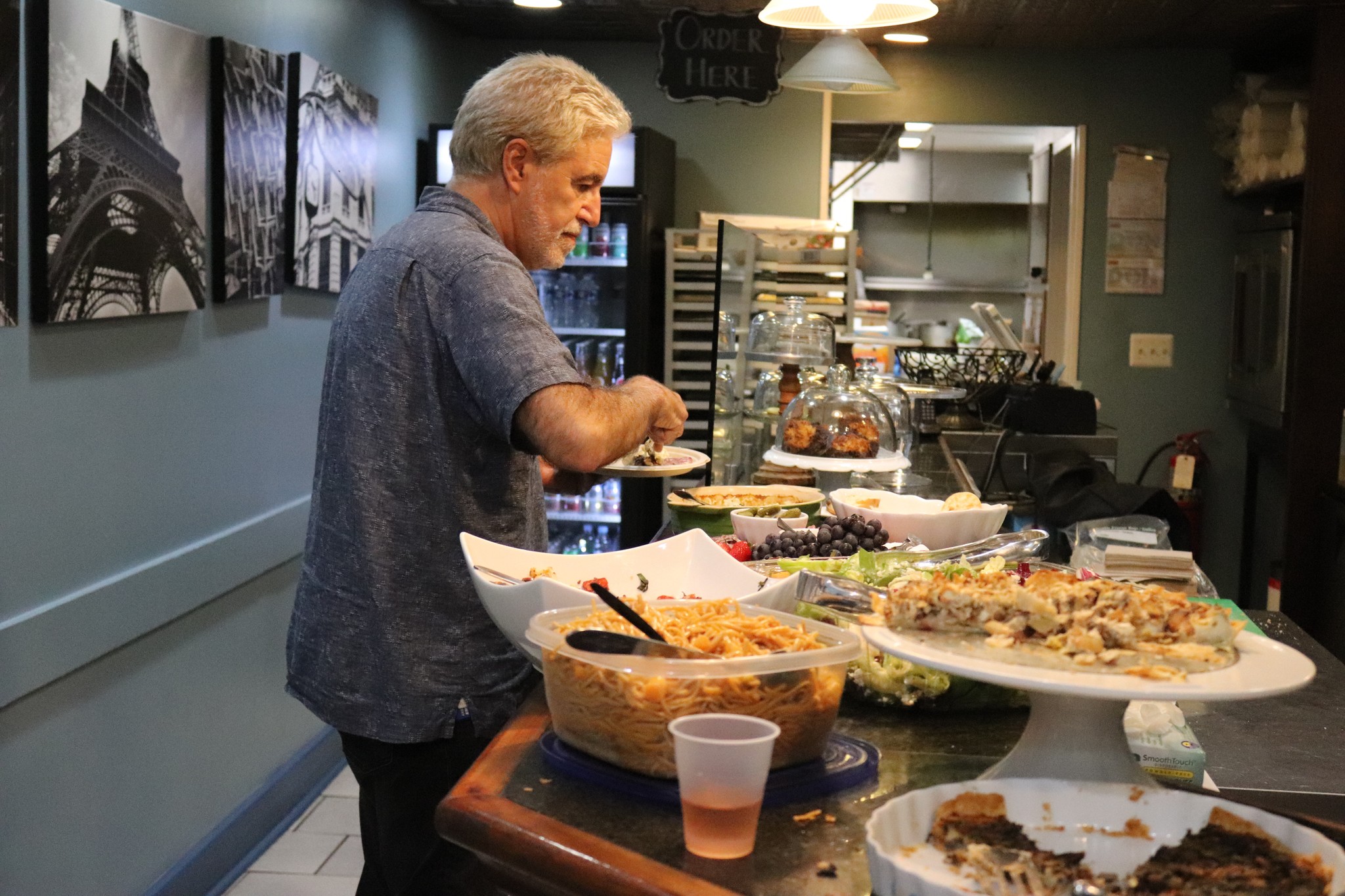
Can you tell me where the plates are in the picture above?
[590,444,711,477]
[459,528,807,675]
[864,778,1345,896]
[860,599,1317,700]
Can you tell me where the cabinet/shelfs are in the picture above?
[925,425,1128,492]
[653,199,734,490]
[820,111,1063,403]
[699,202,871,481]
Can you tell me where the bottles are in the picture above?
[561,339,625,386]
[530,270,598,328]
[644,438,656,462]
[549,524,620,555]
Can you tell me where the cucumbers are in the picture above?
[736,504,801,519]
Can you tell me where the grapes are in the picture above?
[752,514,890,561]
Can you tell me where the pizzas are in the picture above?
[929,791,1335,896]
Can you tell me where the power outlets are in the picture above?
[1129,333,1172,370]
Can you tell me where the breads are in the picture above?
[939,491,982,512]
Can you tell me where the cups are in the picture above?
[667,713,782,860]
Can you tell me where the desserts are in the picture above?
[782,417,880,459]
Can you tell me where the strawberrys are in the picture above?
[715,539,755,563]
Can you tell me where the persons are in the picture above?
[285,51,688,896]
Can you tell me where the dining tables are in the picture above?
[417,498,1345,896]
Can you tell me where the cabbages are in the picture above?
[767,550,1007,703]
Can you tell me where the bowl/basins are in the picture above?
[779,528,819,537]
[829,488,1008,550]
[904,319,957,348]
[667,484,826,546]
[524,599,862,778]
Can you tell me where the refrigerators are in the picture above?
[428,121,678,555]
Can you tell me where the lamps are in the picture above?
[743,1,949,94]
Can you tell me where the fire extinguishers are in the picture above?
[1136,428,1213,562]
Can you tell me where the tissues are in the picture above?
[1123,700,1206,790]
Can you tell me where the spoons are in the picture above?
[565,627,812,693]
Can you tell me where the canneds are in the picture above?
[543,477,620,515]
[569,222,628,260]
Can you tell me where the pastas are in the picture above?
[544,597,846,779]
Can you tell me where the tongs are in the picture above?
[753,556,850,576]
[797,569,887,619]
[914,529,1049,567]
